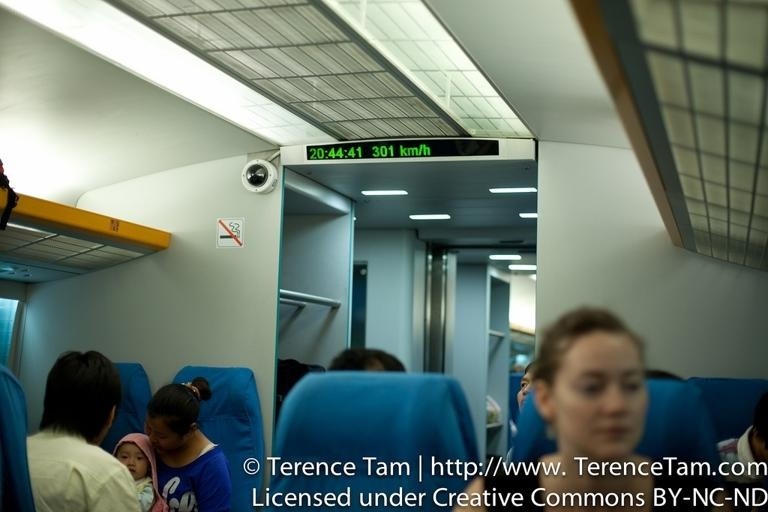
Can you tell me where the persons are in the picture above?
[325,345,409,373]
[24,347,145,512]
[449,302,741,512]
[111,430,164,512]
[143,376,235,512]
[504,358,539,472]
[713,383,767,484]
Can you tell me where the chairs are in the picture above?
[0,359,767,512]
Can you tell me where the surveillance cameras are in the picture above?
[239,158,277,192]
